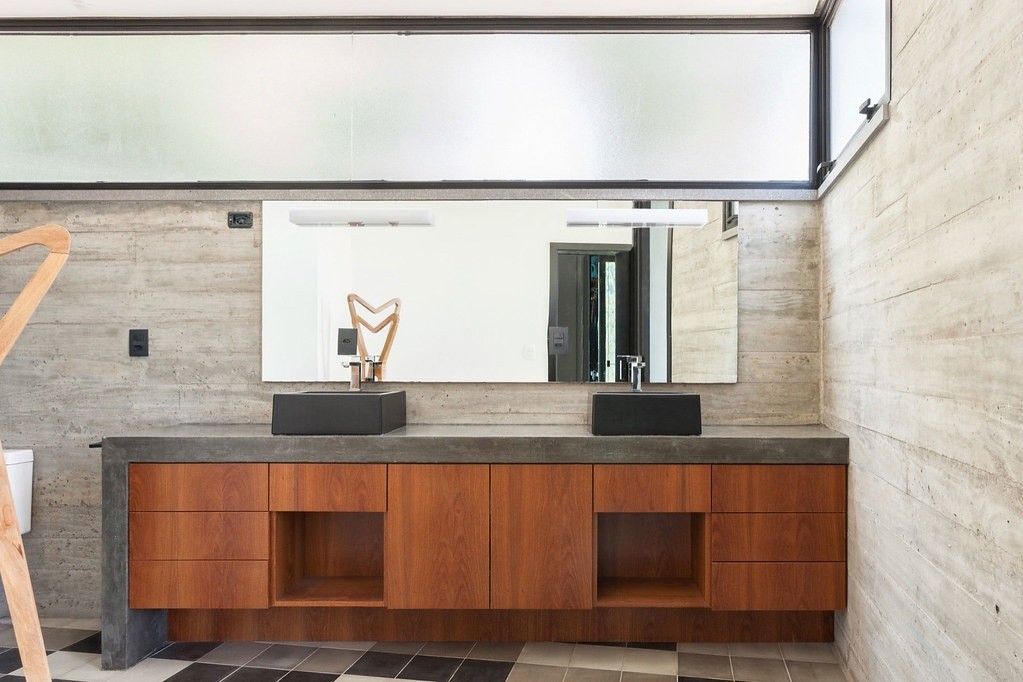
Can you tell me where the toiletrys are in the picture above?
[364,356,373,382]
[373,355,383,381]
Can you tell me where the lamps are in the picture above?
[289,208,435,226]
[566,208,708,229]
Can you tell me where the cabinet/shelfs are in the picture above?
[710,463,847,612]
[127,463,268,611]
[592,463,710,610]
[267,463,386,608]
[386,463,593,611]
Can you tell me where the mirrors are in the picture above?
[261,201,738,384]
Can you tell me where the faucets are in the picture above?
[342,356,361,391]
[632,361,646,392]
[626,356,642,381]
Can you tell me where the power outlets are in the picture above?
[129,330,149,356]
[337,327,357,356]
[547,327,568,355]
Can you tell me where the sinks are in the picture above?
[592,390,702,437]
[272,391,406,435]
